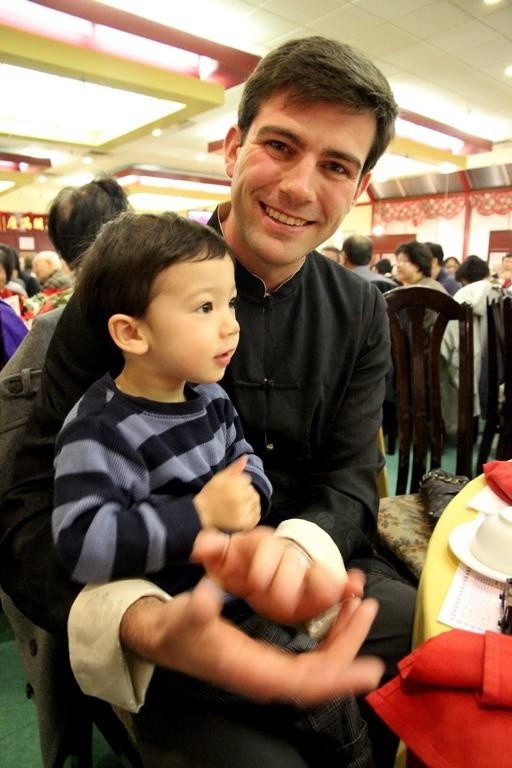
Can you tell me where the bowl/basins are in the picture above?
[474,507,512,575]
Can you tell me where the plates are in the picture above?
[448,517,509,589]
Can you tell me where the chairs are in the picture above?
[476,293,512,477]
[381,286,475,494]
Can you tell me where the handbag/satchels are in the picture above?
[419,468,469,525]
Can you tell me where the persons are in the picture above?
[1,35,419,768]
[51,209,376,766]
[0,177,134,370]
[321,236,511,433]
